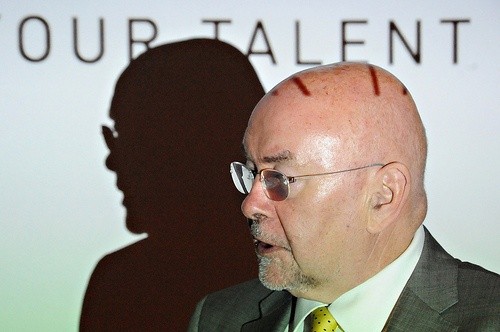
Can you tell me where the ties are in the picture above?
[306,307,339,332]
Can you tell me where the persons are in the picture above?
[187,62,500,332]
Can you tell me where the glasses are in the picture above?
[230,162,385,201]
